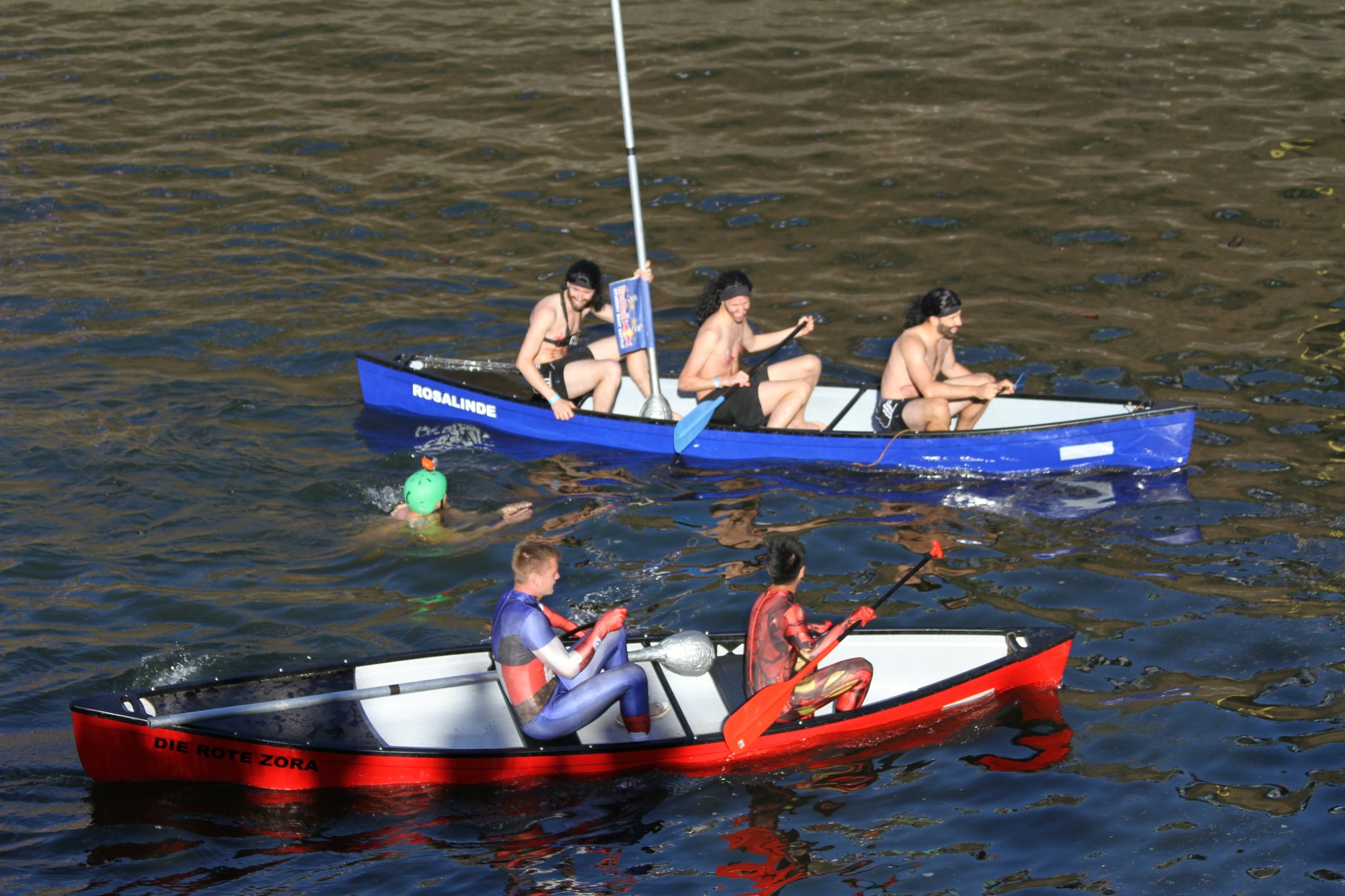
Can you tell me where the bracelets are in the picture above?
[548,393,561,405]
[714,376,721,389]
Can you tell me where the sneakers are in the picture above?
[615,700,671,728]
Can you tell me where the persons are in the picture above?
[743,533,877,725]
[491,540,652,742]
[391,469,447,520]
[874,286,1013,433]
[678,269,828,430]
[515,258,684,421]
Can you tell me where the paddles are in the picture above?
[996,366,1031,394]
[672,319,809,456]
[557,619,598,641]
[719,539,946,755]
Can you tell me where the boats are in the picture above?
[71,626,1080,791]
[355,345,1198,476]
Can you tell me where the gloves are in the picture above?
[812,620,833,634]
[849,605,877,627]
[595,607,626,637]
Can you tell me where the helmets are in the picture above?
[403,469,448,515]
